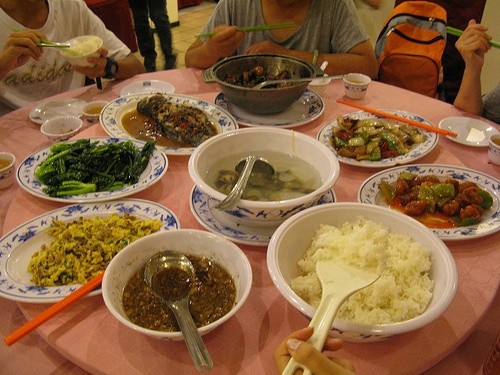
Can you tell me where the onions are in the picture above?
[334,115,423,152]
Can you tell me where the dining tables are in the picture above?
[0,67,500,375]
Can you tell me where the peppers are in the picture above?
[334,133,401,161]
[379,173,494,227]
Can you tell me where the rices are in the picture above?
[290,216,432,325]
[61,39,101,56]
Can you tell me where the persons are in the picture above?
[84,0,138,53]
[185,0,379,81]
[128,0,176,72]
[394,0,486,105]
[273,327,357,375]
[0,0,146,117]
[453,19,500,125]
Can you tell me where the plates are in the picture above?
[0,197,182,302]
[16,136,169,202]
[29,97,87,124]
[119,79,175,97]
[212,91,323,130]
[357,163,500,240]
[316,109,438,168]
[190,183,337,246]
[437,116,497,147]
[99,91,236,154]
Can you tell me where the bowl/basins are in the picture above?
[101,229,253,341]
[202,55,324,116]
[189,126,343,223]
[267,202,459,345]
[61,35,103,68]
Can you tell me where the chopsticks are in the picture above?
[194,20,296,40]
[336,98,458,139]
[11,27,81,57]
[5,271,105,347]
[445,25,499,50]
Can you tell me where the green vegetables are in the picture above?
[34,137,158,195]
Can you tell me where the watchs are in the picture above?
[96,57,118,90]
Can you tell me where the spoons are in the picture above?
[253,75,347,89]
[142,250,214,371]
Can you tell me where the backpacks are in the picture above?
[373,2,447,103]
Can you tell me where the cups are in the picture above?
[0,152,17,190]
[343,73,371,100]
[39,115,83,143]
[82,100,109,125]
[488,134,500,165]
